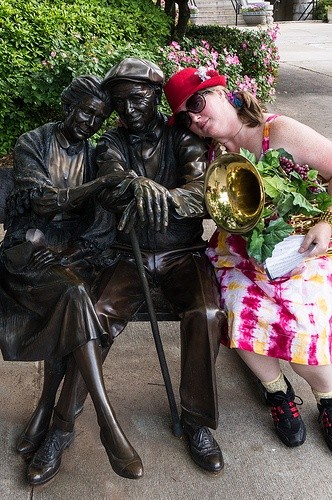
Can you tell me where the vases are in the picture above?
[177,13,198,23]
[242,11,267,26]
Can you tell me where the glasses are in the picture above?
[174,91,208,129]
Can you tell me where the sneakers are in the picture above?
[317,397,332,450]
[264,374,306,448]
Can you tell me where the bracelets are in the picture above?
[311,210,332,227]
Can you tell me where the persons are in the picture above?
[163,68,332,450]
[0,57,226,485]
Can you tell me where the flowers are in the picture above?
[242,3,270,12]
[188,3,200,13]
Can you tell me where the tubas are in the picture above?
[204,151,318,236]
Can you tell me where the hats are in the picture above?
[163,67,225,127]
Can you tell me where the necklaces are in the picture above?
[222,123,244,146]
